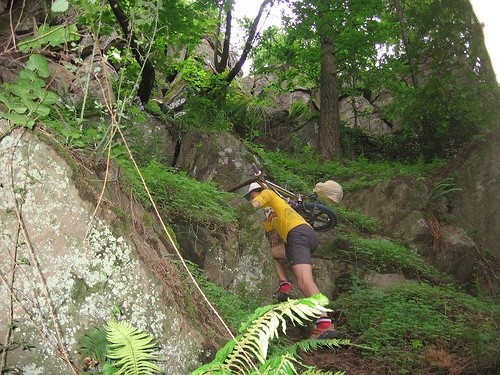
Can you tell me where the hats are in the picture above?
[243,183,261,197]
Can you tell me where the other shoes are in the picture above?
[308,317,337,340]
[273,279,295,303]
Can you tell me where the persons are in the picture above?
[243,182,338,339]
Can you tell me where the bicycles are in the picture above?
[225,162,337,232]
